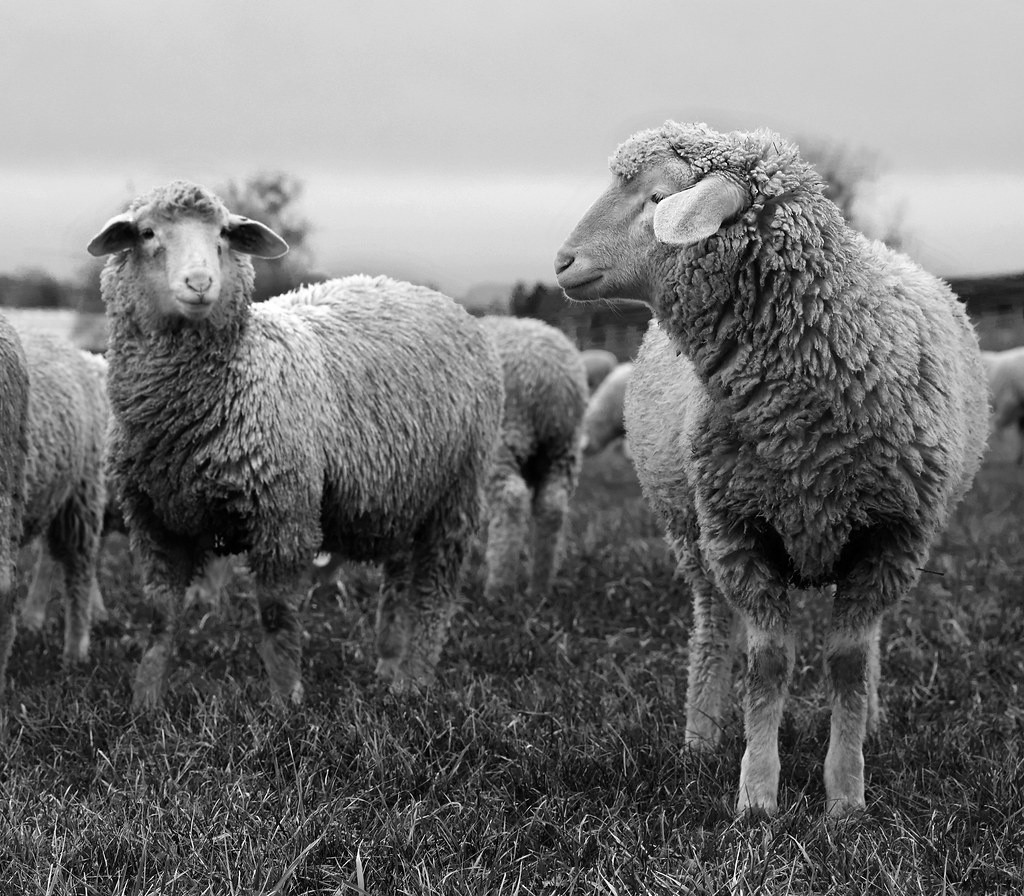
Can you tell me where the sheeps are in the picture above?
[1,121,1024,831]
